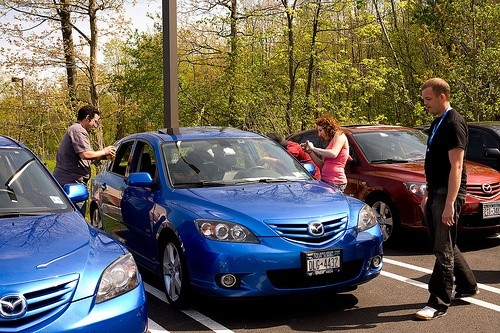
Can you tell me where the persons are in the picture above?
[300,118,349,193]
[414,77,480,321]
[53,105,116,219]
[257,132,321,180]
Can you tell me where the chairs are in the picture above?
[141,152,213,172]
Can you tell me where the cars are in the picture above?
[0,135,147,333]
[286,125,500,250]
[465,121,500,172]
[90,126,386,313]
